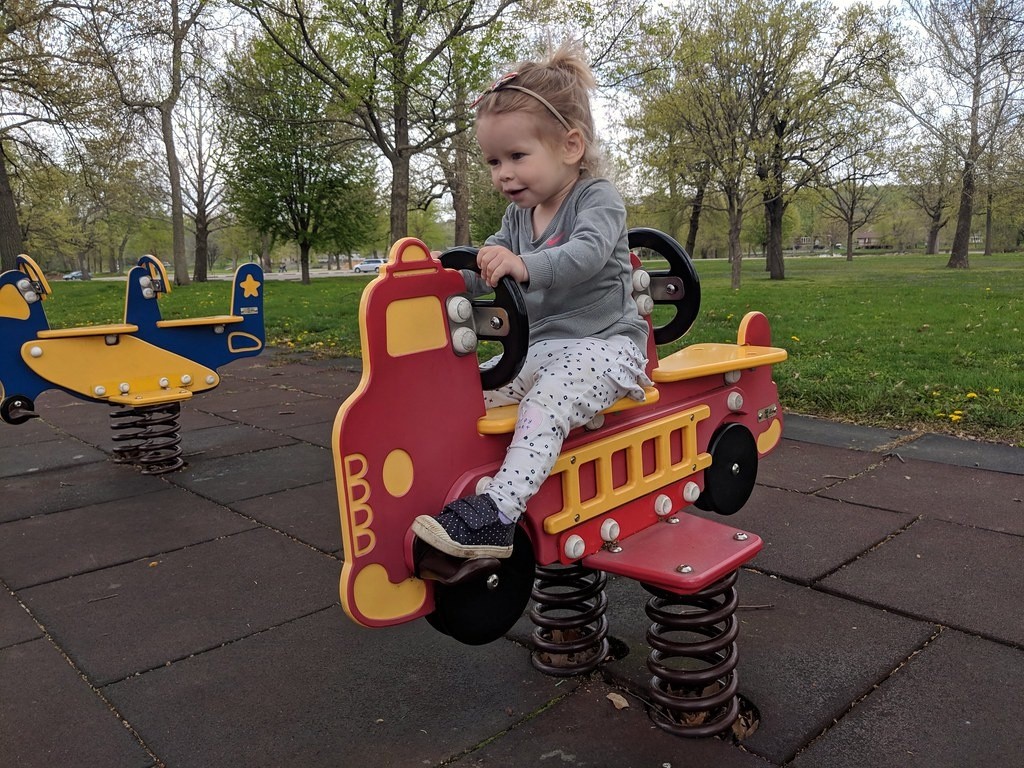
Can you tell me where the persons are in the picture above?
[279,263,287,273]
[411,39,655,558]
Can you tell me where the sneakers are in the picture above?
[411,493,515,558]
